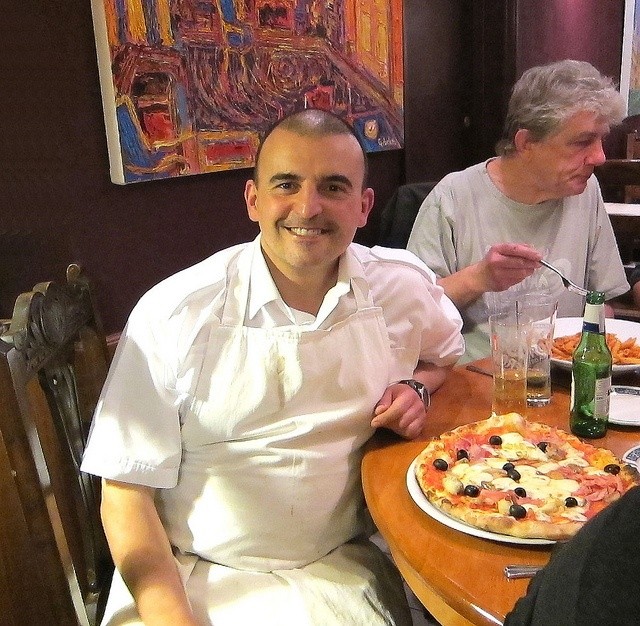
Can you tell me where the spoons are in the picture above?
[466,365,547,388]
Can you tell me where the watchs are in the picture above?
[396,379,431,414]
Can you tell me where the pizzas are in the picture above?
[414,412,639,540]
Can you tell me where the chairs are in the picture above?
[7,264,113,624]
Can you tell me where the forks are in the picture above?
[534,258,591,296]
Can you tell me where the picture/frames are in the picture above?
[89,1,407,186]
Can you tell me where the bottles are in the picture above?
[569,291,614,438]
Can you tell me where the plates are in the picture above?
[532,317,640,375]
[581,385,639,426]
[405,451,558,546]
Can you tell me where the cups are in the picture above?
[488,313,533,420]
[516,293,559,405]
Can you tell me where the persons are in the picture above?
[629,265,640,308]
[80,108,466,626]
[405,59,631,369]
